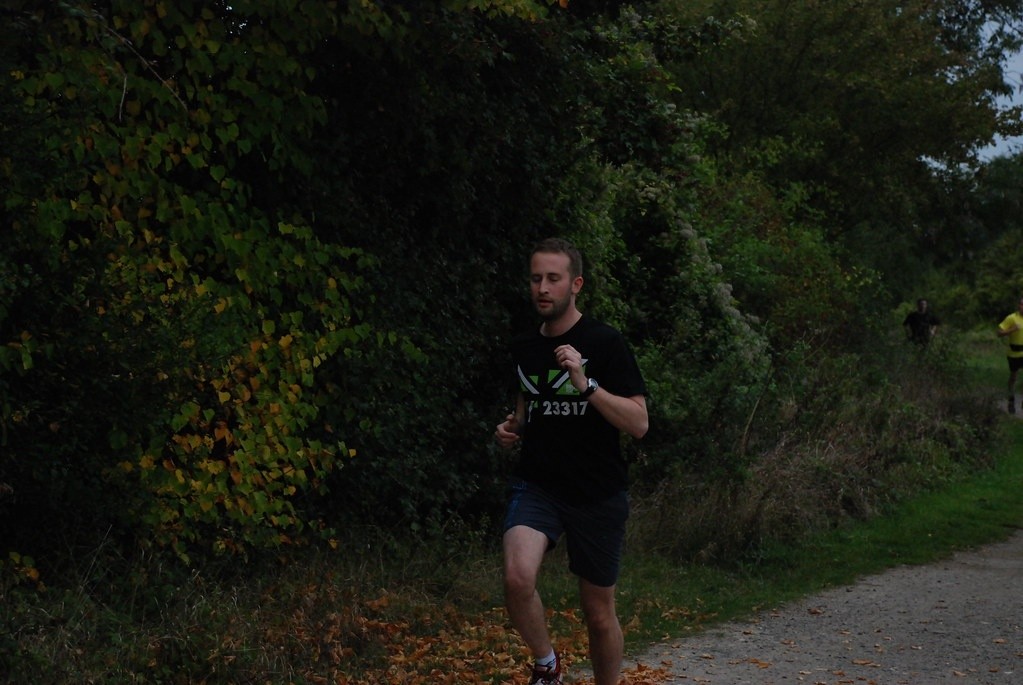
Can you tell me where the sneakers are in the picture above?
[528,647,563,685]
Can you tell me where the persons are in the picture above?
[494,238,652,685]
[902,296,939,353]
[996,296,1023,414]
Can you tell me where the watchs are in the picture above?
[579,377,598,399]
